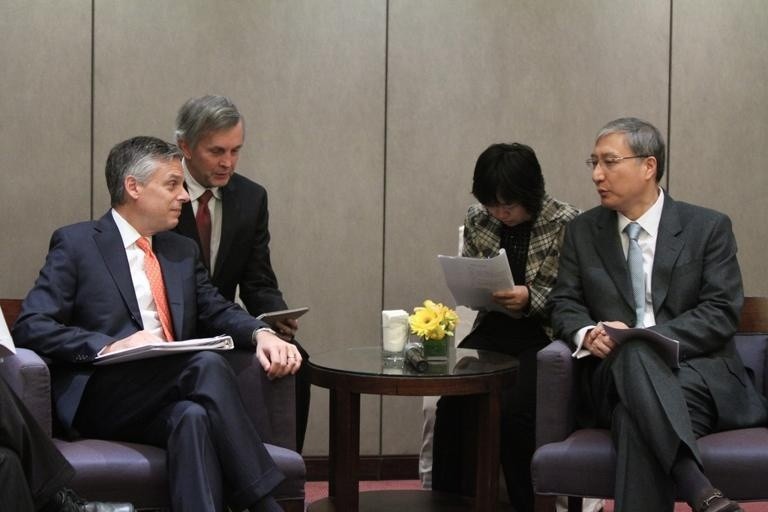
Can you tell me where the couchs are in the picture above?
[530,295,768,512]
[1,344,304,511]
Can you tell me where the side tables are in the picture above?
[307,346,518,512]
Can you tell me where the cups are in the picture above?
[380,324,410,362]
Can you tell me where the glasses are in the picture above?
[586,154,646,169]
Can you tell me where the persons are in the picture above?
[0,378,134,512]
[167,92,312,455]
[431,141,585,512]
[16,136,287,512]
[550,117,768,512]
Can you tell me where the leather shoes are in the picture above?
[690,488,743,512]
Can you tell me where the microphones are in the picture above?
[402,341,429,372]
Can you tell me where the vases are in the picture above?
[422,329,454,359]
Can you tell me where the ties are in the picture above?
[135,237,176,343]
[195,189,212,276]
[625,223,646,330]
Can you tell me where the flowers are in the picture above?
[408,299,458,359]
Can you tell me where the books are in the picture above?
[256,306,310,332]
